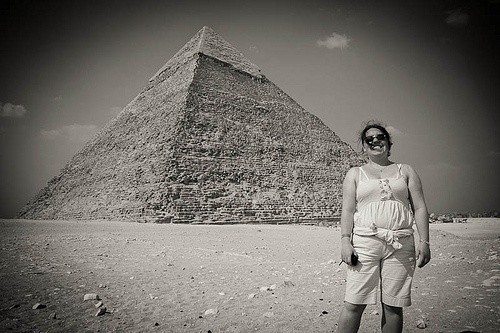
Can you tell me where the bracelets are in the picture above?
[339,234,350,238]
[418,238,431,245]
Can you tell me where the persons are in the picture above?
[333,118,431,333]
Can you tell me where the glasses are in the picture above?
[363,134,388,142]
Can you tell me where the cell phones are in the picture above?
[351,254,357,266]
